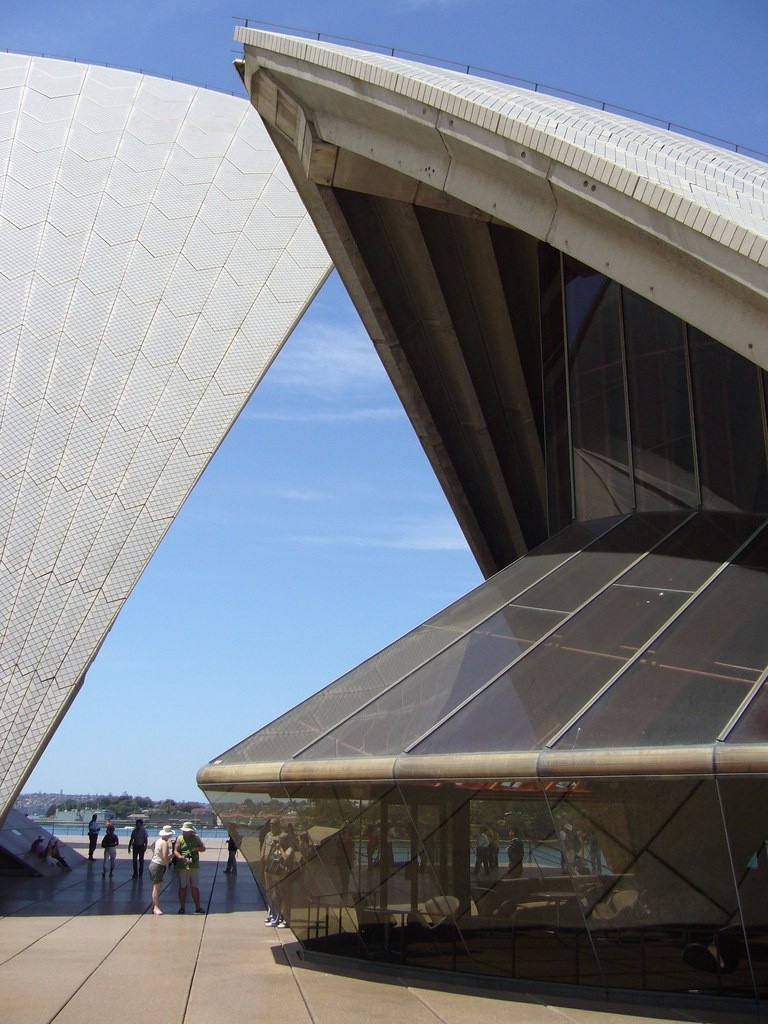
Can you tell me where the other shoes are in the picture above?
[223,870,231,873]
[195,907,205,913]
[139,873,143,877]
[265,922,278,927]
[264,918,270,922]
[109,874,113,877]
[67,867,72,871]
[179,908,185,914]
[133,872,138,877]
[277,923,289,928]
[102,874,105,878]
[153,910,163,915]
[89,857,96,862]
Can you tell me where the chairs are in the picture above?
[351,868,715,946]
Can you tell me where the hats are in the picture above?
[39,835,45,840]
[159,825,176,836]
[563,824,573,831]
[180,822,196,832]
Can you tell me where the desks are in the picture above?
[528,892,579,932]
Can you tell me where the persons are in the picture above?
[260,817,280,922]
[128,820,149,879]
[367,821,396,867]
[102,825,118,877]
[560,821,602,874]
[148,824,174,915]
[336,827,355,898]
[266,832,295,929]
[475,824,500,874]
[223,823,243,873]
[32,836,72,872]
[506,827,524,878]
[259,820,309,862]
[88,814,101,861]
[173,822,207,914]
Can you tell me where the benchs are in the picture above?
[467,870,668,921]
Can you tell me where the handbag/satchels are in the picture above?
[270,854,293,874]
